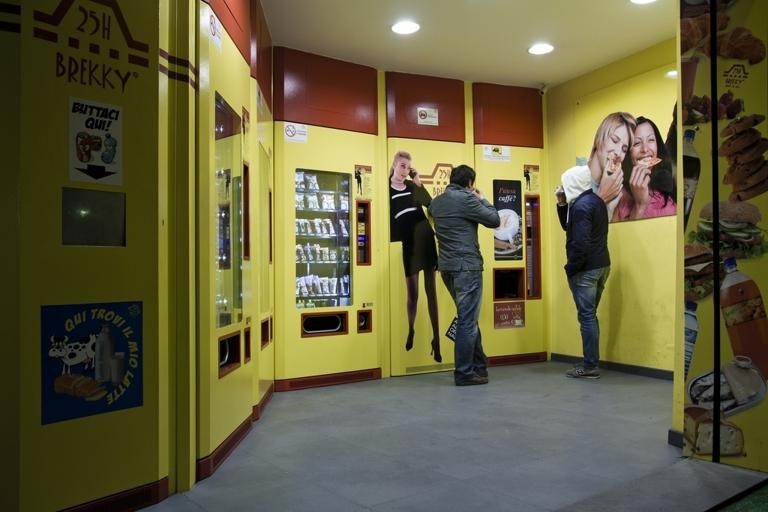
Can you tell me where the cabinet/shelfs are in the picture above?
[683,128,702,235]
[684,299,699,384]
[719,257,768,385]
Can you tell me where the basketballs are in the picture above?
[718,114,768,202]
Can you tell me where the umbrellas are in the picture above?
[454,374,489,386]
[475,368,489,377]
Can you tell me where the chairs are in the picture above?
[566,365,601,379]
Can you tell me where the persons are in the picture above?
[426,164,501,387]
[388,150,443,363]
[553,164,612,380]
[524,168,531,191]
[575,110,638,224]
[610,116,677,224]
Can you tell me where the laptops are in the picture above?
[684,407,746,457]
[701,27,766,65]
[681,14,730,55]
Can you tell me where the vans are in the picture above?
[431,340,442,363]
[406,331,414,352]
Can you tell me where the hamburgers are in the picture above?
[687,201,768,261]
[684,245,728,300]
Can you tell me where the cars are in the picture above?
[681,57,698,110]
